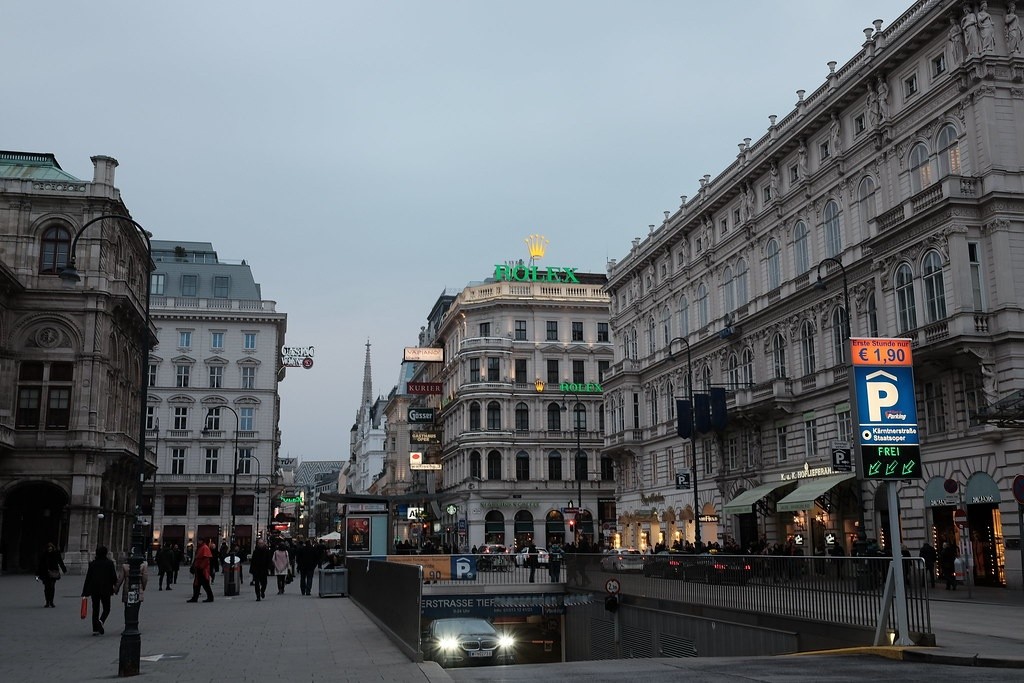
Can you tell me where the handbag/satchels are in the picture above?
[48,570,61,580]
[285,569,293,584]
[80,596,88,618]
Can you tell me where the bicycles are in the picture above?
[474,552,516,573]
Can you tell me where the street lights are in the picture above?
[57,214,153,678]
[236,455,260,547]
[146,415,160,564]
[664,337,702,554]
[200,405,238,597]
[558,390,584,528]
[812,257,870,555]
[253,476,270,550]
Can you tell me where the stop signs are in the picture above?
[955,509,967,529]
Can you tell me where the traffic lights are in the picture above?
[604,595,618,611]
[570,521,574,532]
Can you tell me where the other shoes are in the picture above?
[203,598,214,602]
[186,599,198,602]
[49,602,55,607]
[256,598,260,601]
[96,620,104,635]
[306,593,311,596]
[277,591,283,595]
[166,586,172,591]
[302,593,305,595]
[261,591,265,598]
[44,605,49,608]
[158,587,162,591]
[93,628,97,632]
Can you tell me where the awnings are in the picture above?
[776,473,856,512]
[723,480,798,516]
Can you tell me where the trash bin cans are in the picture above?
[223,555,241,595]
[320,569,347,597]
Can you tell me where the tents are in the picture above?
[317,531,341,544]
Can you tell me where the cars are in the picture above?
[600,548,644,574]
[682,548,751,585]
[423,619,517,668]
[514,546,550,569]
[477,543,509,554]
[643,549,691,580]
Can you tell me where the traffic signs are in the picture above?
[853,363,923,480]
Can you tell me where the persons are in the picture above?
[34,542,67,608]
[563,537,615,585]
[155,539,243,603]
[113,546,148,601]
[919,539,936,589]
[941,541,957,590]
[82,546,118,636]
[250,538,341,596]
[393,536,464,555]
[644,537,913,588]
[272,544,290,594]
[527,539,539,583]
[249,539,273,601]
[973,531,990,576]
[353,522,364,535]
[549,539,565,583]
[472,543,523,554]
